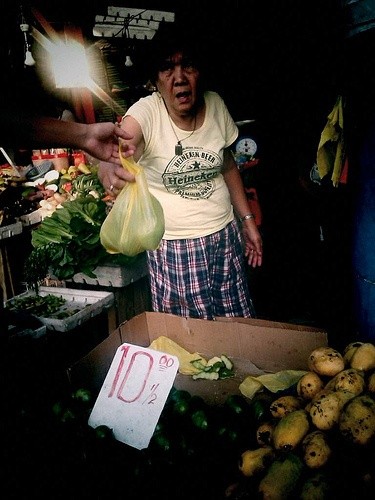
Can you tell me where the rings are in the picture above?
[109,185,114,190]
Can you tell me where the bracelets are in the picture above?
[238,213,256,222]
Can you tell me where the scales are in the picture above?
[234,119,259,168]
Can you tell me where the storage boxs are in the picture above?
[63,256,149,287]
[65,311,327,412]
[7,286,113,331]
[4,322,46,338]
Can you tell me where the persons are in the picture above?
[97,48,264,321]
[0,110,137,166]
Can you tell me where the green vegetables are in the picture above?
[26,170,137,282]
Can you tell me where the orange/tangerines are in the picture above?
[38,383,271,500]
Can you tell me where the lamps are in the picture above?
[20,24,36,66]
[122,28,133,66]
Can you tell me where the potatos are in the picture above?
[236,340,375,500]
